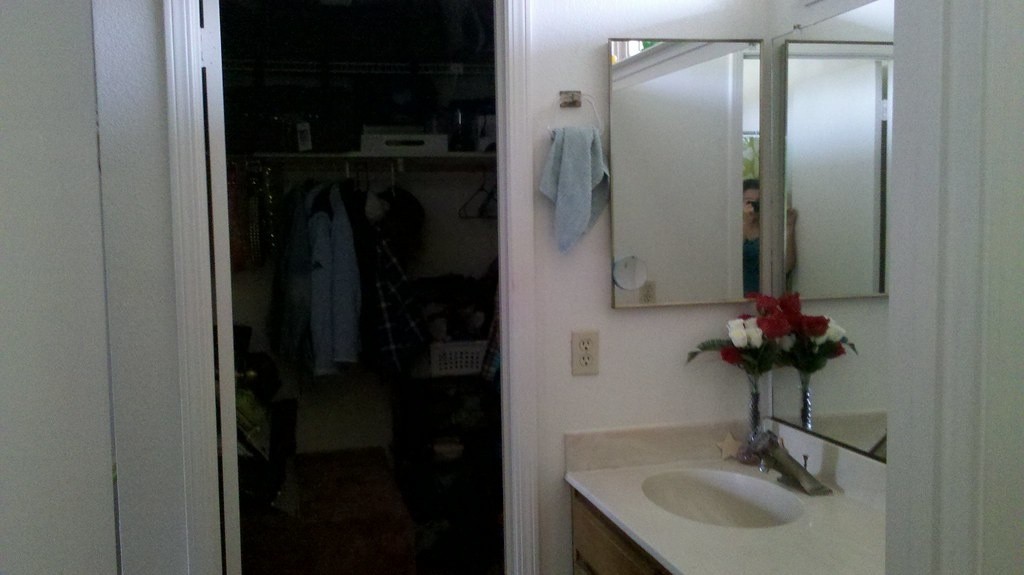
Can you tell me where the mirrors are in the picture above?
[777,39,897,299]
[768,0,915,464]
[608,37,765,310]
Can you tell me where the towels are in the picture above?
[537,124,611,255]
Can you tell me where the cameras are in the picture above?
[746,199,760,213]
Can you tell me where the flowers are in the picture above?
[778,290,860,377]
[685,310,794,393]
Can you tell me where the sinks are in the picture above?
[641,470,805,528]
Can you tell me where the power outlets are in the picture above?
[570,327,600,376]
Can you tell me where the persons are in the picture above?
[743,177,798,298]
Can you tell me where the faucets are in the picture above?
[749,429,833,496]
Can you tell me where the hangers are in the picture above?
[307,161,399,199]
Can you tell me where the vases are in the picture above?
[798,372,814,430]
[737,373,764,466]
